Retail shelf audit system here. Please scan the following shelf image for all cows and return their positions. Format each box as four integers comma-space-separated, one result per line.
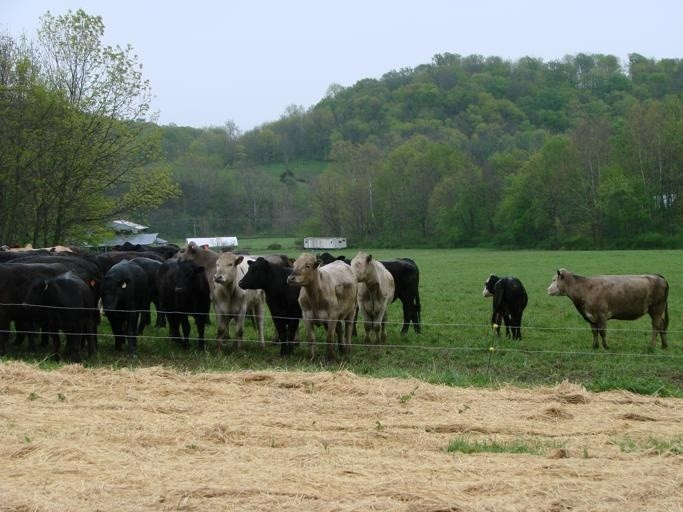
482, 273, 528, 341
547, 267, 669, 353
0, 236, 422, 364
